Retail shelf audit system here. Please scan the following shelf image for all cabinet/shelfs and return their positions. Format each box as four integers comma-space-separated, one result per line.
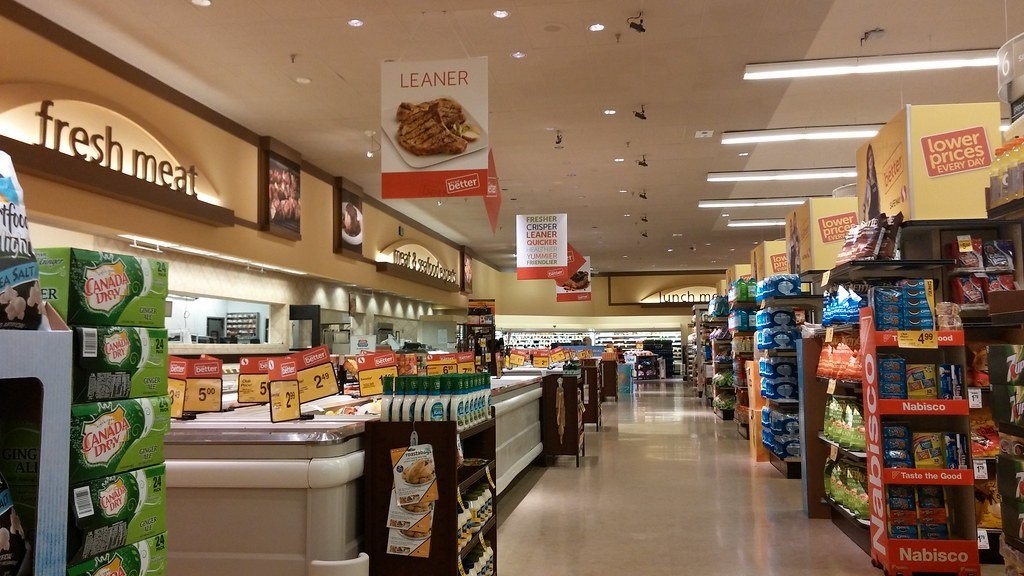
595, 334, 682, 359
685, 197, 1024, 576
225, 312, 259, 339
540, 372, 585, 468
358, 406, 497, 576
563, 364, 602, 432
635, 354, 660, 380
599, 359, 619, 402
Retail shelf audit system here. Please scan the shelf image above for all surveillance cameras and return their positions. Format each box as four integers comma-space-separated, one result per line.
642, 234, 648, 237
642, 218, 648, 223
638, 162, 648, 166
634, 112, 647, 121
631, 22, 646, 33
640, 194, 647, 199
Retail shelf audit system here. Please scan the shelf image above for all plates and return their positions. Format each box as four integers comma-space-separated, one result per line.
398, 529, 431, 540
381, 96, 488, 169
342, 202, 363, 245
399, 505, 431, 514
401, 462, 436, 487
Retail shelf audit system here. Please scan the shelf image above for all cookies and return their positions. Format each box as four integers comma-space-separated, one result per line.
906, 280, 921, 324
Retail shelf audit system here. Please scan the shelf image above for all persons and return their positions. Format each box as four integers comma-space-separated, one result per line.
788, 212, 801, 274
862, 144, 881, 223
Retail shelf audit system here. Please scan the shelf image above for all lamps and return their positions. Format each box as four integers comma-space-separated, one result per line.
634, 154, 648, 167
707, 166, 858, 182
633, 104, 647, 120
697, 194, 832, 210
641, 216, 649, 222
720, 122, 886, 145
632, 104, 646, 120
638, 192, 648, 199
642, 232, 648, 237
742, 46, 999, 82
556, 130, 563, 144
726, 217, 786, 228
627, 10, 647, 35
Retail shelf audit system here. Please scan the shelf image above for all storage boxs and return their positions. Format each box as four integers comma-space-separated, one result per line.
66, 531, 168, 576
998, 535, 1024, 569
991, 384, 1024, 427
67, 462, 167, 567
1004, 558, 1024, 576
70, 395, 171, 484
725, 263, 751, 287
995, 453, 1024, 503
750, 240, 789, 281
855, 102, 1001, 221
784, 197, 859, 275
72, 327, 169, 403
32, 247, 169, 328
716, 280, 726, 297
1000, 495, 1024, 542
986, 343, 1024, 384
331, 331, 349, 354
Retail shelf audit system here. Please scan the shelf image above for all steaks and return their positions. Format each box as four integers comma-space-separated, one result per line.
396, 100, 468, 156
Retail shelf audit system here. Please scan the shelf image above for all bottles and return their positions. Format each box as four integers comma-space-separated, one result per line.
458, 482, 494, 576
380, 371, 492, 432
